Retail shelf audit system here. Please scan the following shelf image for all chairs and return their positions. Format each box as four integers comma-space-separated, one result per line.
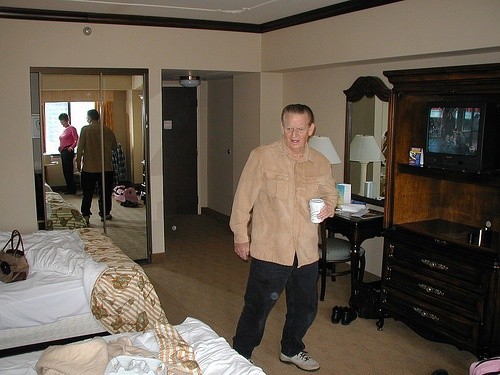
318, 223, 367, 301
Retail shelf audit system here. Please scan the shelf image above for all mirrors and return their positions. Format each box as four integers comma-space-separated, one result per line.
29, 66, 153, 266
337, 75, 392, 206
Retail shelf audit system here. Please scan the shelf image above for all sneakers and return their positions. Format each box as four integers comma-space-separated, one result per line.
279, 351, 321, 371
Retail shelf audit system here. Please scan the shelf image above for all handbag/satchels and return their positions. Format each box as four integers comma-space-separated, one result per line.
0, 230, 30, 284
468, 356, 500, 375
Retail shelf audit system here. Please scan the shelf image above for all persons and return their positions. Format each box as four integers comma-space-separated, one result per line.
76, 109, 117, 225
58, 113, 79, 194
230, 102, 338, 372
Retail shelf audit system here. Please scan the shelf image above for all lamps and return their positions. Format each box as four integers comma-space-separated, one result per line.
350, 134, 385, 196
309, 134, 343, 165
179, 76, 201, 88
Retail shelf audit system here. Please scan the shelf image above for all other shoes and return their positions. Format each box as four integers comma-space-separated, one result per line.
331, 306, 357, 325
62, 188, 74, 194
101, 213, 112, 222
120, 200, 138, 208
83, 215, 90, 224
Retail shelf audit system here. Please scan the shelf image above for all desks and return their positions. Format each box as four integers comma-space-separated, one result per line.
325, 209, 385, 296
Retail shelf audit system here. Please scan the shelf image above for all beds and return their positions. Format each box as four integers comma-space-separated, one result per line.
0, 314, 265, 375
0, 228, 170, 350
44, 191, 89, 230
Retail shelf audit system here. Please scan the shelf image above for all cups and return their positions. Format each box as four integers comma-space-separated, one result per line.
50, 156, 59, 164
309, 198, 325, 224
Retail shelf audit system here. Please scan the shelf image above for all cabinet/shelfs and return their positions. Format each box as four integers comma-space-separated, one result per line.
374, 62, 500, 362
